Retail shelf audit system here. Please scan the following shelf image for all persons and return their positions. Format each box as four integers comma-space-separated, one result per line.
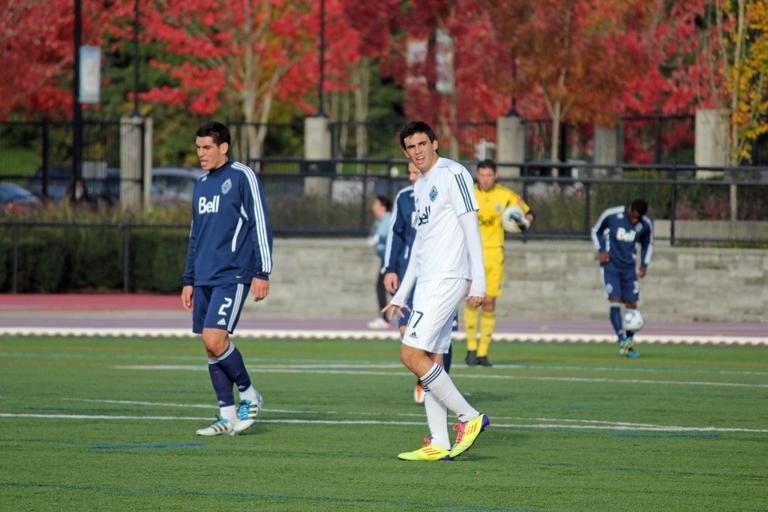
590, 197, 654, 358
383, 153, 453, 405
463, 156, 537, 368
179, 121, 273, 437
380, 120, 492, 462
368, 195, 400, 330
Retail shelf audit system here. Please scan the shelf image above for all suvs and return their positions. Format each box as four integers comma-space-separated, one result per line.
524, 159, 594, 196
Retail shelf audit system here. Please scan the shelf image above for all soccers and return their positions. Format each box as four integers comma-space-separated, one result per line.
622, 308, 646, 334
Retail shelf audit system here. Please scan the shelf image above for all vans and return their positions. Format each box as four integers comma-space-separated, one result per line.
151, 166, 205, 202
31, 167, 121, 200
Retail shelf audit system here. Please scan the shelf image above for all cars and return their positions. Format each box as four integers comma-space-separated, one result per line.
0, 182, 41, 211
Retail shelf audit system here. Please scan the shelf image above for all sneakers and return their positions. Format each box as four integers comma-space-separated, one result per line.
619, 336, 634, 355
413, 381, 425, 404
195, 415, 239, 436
476, 356, 492, 367
625, 349, 641, 359
449, 412, 489, 460
367, 318, 391, 329
465, 351, 480, 365
234, 389, 263, 433
397, 438, 455, 460
